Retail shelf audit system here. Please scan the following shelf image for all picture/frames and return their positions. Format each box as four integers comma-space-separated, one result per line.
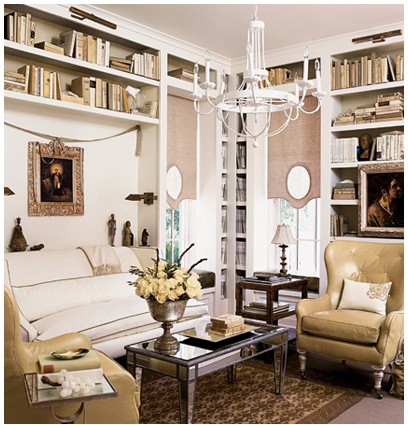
357, 163, 404, 238
27, 142, 84, 216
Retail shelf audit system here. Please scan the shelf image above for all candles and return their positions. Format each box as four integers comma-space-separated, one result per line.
314, 59, 321, 93
205, 48, 211, 82
220, 70, 226, 94
294, 72, 299, 101
303, 45, 311, 81
193, 61, 199, 93
245, 41, 253, 74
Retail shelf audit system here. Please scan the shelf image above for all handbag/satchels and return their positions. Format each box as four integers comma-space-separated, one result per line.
383, 336, 404, 400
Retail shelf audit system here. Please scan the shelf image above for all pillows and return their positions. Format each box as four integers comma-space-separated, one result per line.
336, 278, 392, 316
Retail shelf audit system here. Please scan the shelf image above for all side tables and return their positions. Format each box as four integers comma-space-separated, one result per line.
23, 367, 118, 424
236, 278, 309, 345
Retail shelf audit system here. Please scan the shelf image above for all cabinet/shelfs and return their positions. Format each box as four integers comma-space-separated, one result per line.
221, 63, 254, 314
254, 44, 326, 95
4, 4, 160, 125
161, 40, 222, 101
326, 21, 404, 237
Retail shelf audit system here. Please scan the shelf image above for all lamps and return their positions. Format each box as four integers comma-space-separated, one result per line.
191, 5, 325, 147
272, 223, 296, 280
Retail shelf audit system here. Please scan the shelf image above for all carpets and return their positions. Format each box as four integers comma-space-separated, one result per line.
115, 346, 374, 424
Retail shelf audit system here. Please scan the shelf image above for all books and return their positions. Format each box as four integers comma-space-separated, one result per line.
208, 313, 246, 336
220, 176, 246, 202
243, 299, 289, 314
168, 67, 201, 83
221, 239, 246, 266
223, 143, 246, 167
222, 208, 246, 233
331, 138, 358, 162
266, 69, 292, 86
332, 52, 404, 90
332, 187, 356, 199
330, 214, 348, 235
377, 130, 404, 160
4, 65, 159, 116
222, 275, 242, 295
4, 9, 159, 79
38, 350, 100, 372
333, 91, 404, 125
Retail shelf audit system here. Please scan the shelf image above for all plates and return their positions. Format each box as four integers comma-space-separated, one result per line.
178, 326, 261, 343
50, 348, 89, 360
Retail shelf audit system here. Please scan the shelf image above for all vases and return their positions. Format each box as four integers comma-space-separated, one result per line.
142, 295, 189, 348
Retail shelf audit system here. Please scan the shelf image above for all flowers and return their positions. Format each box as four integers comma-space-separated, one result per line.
126, 242, 207, 303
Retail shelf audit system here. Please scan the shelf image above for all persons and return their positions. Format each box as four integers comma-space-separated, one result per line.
51, 168, 64, 199
124, 221, 132, 247
368, 177, 400, 227
10, 217, 28, 251
108, 214, 117, 247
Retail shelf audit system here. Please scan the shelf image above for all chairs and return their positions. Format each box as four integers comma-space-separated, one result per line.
4, 287, 141, 424
294, 241, 404, 399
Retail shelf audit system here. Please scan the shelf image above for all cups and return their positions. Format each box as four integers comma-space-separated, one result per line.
195, 318, 207, 337
